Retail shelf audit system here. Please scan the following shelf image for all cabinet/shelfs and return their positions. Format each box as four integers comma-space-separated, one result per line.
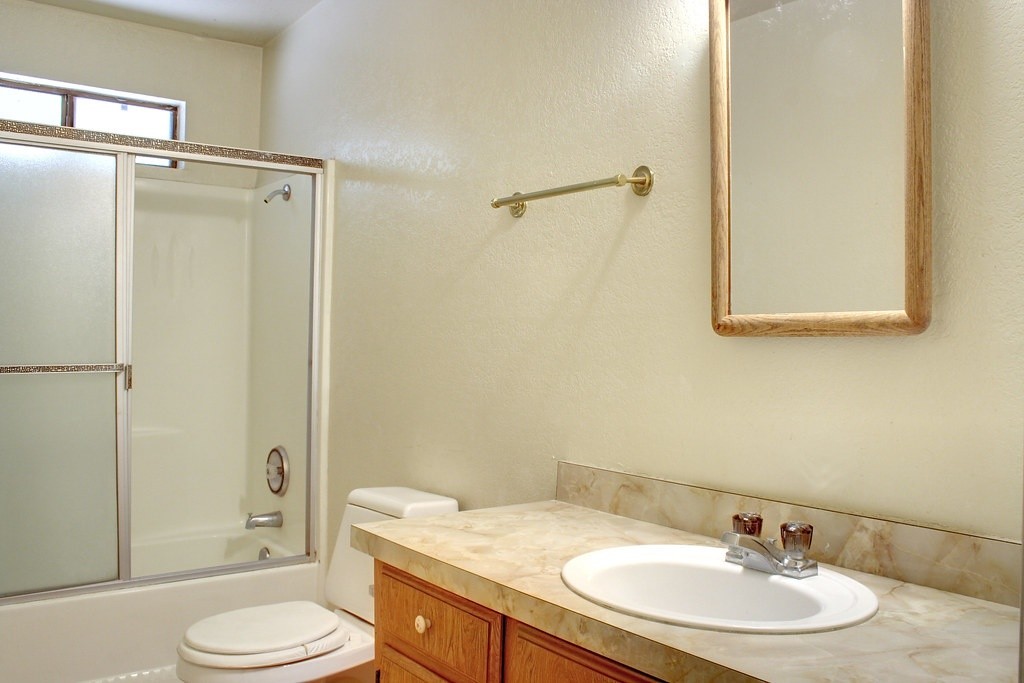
374, 559, 667, 683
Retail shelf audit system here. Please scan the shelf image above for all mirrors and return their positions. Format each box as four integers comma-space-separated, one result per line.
709, 0, 931, 338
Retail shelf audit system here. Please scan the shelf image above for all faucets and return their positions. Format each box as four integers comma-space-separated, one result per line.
245, 510, 283, 529
720, 511, 818, 580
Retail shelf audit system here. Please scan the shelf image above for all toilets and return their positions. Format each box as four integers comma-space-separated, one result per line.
175, 486, 460, 683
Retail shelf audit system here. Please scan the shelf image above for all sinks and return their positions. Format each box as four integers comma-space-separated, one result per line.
560, 545, 880, 634
